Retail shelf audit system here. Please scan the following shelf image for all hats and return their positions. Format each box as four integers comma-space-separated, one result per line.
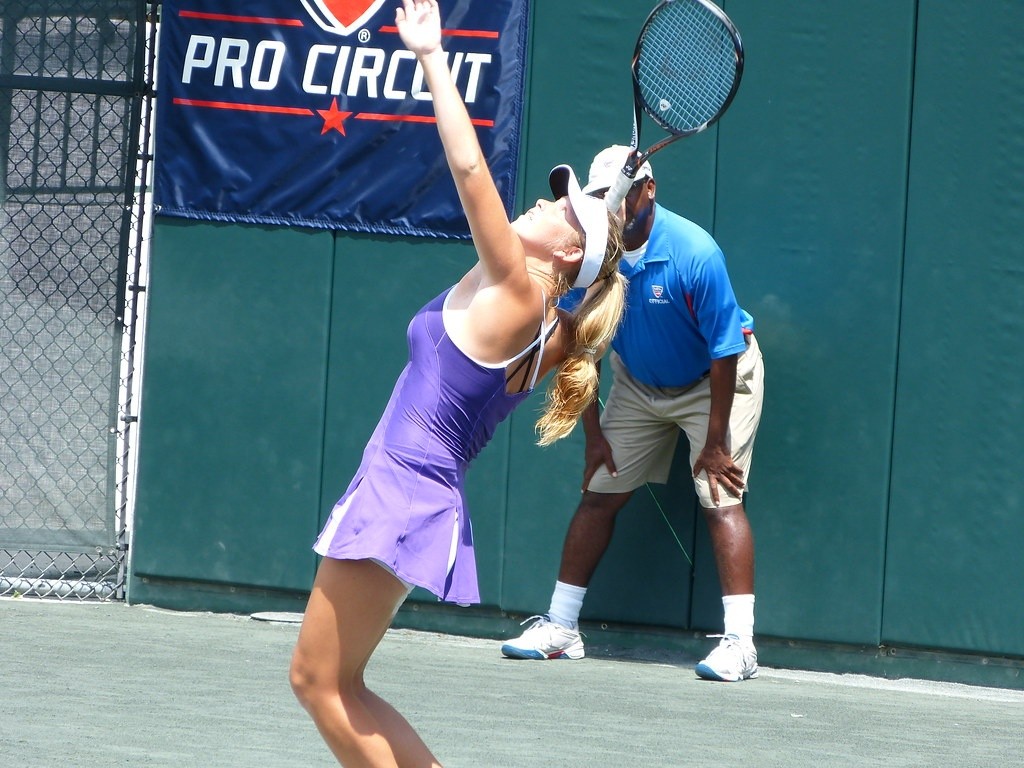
548, 164, 609, 288
582, 144, 653, 194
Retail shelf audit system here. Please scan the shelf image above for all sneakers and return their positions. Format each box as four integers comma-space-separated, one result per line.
501, 616, 586, 660
695, 634, 759, 682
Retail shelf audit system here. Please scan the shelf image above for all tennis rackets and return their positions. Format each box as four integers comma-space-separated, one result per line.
603, 0, 746, 214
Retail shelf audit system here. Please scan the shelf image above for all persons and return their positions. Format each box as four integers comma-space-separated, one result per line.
284, 0, 626, 768
497, 144, 766, 683
